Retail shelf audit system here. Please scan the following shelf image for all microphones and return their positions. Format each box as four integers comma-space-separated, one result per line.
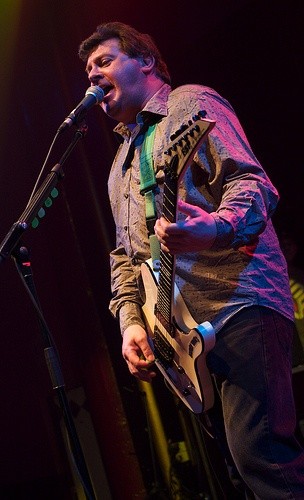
58, 85, 104, 134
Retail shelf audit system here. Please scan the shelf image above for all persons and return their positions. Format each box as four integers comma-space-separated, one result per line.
78, 21, 303, 499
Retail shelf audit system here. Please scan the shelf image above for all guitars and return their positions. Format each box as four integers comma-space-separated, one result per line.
139, 109, 215, 414
